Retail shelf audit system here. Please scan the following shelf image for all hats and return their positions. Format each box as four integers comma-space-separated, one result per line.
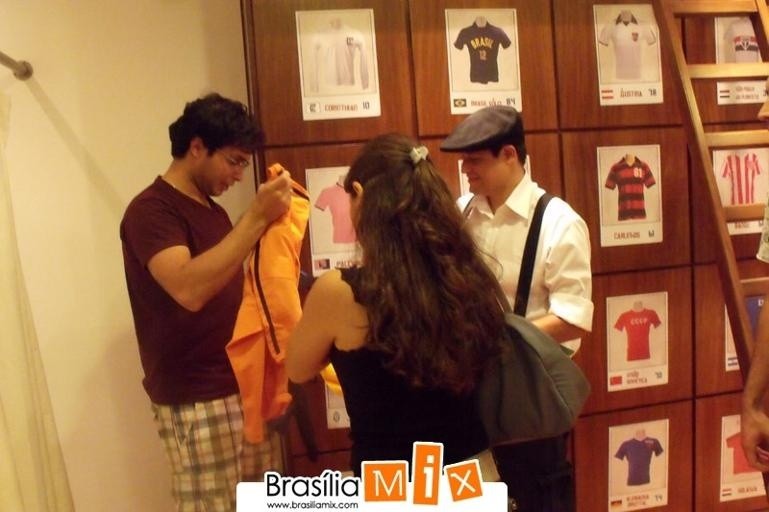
440, 105, 523, 153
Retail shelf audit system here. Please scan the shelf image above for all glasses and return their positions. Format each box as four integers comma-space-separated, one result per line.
217, 148, 250, 167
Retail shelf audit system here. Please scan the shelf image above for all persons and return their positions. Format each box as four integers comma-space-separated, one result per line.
120, 91, 293, 512
740, 76, 769, 472
284, 130, 518, 512
440, 106, 594, 512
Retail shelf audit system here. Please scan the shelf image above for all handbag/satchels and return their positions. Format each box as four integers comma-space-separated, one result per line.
476, 313, 591, 442
